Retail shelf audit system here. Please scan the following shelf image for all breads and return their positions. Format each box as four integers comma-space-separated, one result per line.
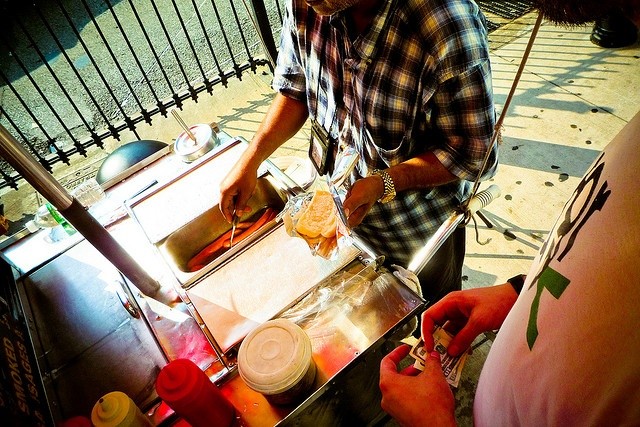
296, 187, 337, 238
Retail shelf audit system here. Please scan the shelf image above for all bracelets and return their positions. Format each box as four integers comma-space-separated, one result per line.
507, 273, 526, 297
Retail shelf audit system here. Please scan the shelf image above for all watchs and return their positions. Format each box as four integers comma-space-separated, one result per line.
366, 168, 398, 206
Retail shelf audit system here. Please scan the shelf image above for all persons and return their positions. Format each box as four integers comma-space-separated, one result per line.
217, 1, 501, 341
378, 0, 639, 426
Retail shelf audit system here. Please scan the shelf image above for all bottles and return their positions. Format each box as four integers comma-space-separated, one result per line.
42, 204, 105, 245
155, 358, 236, 425
91, 389, 151, 426
24, 177, 107, 233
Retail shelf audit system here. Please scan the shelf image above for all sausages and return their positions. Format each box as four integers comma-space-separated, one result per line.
185, 228, 242, 272
223, 204, 280, 249
